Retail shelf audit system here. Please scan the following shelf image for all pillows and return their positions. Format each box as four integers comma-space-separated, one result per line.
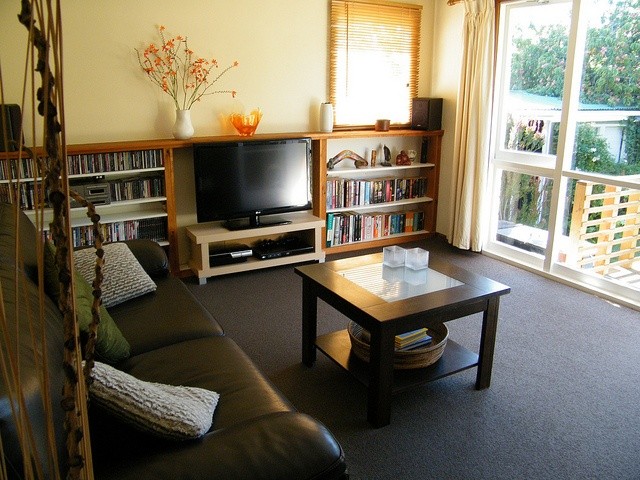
73, 242, 158, 309
44, 237, 133, 364
75, 360, 221, 442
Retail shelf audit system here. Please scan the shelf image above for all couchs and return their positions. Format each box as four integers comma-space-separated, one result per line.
1, 192, 349, 479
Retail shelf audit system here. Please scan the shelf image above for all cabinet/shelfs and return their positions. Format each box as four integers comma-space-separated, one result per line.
313, 129, 444, 255
1, 139, 181, 281
184, 213, 326, 285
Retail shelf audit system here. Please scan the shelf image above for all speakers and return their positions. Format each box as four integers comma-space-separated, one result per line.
411, 97, 443, 129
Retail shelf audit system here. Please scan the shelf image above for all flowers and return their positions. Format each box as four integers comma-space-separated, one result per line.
134, 24, 239, 110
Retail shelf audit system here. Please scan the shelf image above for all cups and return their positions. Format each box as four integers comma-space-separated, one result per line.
406, 247, 430, 271
375, 120, 391, 131
382, 265, 405, 284
405, 266, 428, 286
383, 245, 406, 268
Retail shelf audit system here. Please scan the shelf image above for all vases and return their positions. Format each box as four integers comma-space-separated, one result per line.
320, 103, 334, 132
171, 110, 194, 139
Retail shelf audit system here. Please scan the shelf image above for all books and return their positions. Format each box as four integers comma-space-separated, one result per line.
326, 211, 425, 247
326, 176, 428, 209
0, 149, 163, 180
39, 217, 168, 248
395, 327, 433, 352
0, 175, 165, 210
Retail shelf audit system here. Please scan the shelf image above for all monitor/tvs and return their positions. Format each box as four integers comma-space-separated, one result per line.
191, 137, 313, 232
